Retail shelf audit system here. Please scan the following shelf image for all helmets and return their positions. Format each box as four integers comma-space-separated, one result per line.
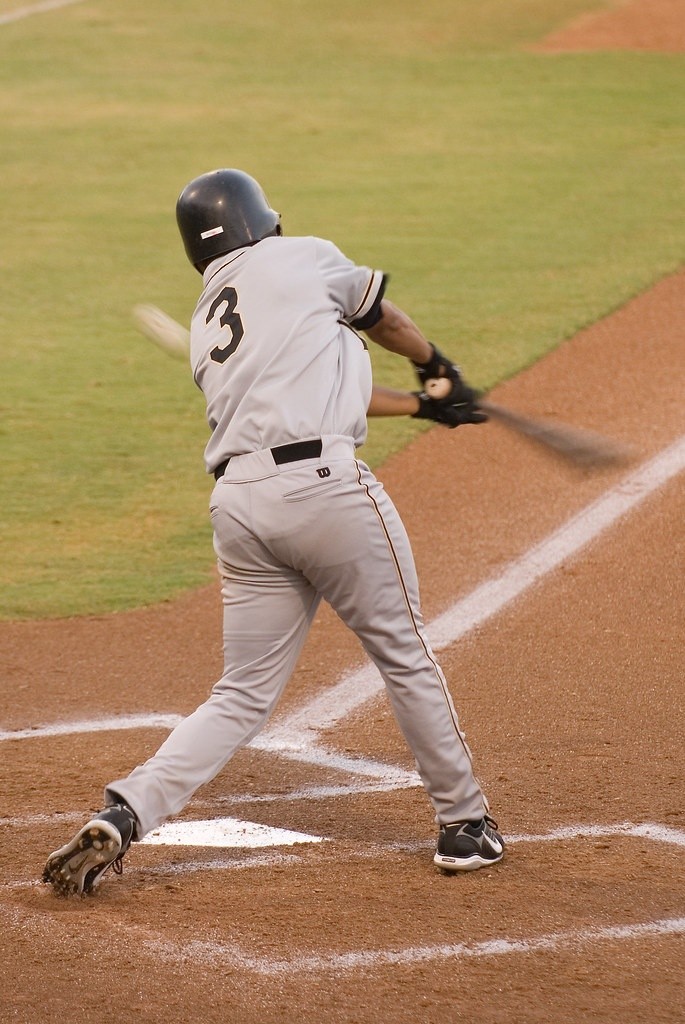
177, 168, 282, 275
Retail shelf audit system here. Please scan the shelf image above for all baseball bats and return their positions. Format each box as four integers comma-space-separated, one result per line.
425, 373, 620, 475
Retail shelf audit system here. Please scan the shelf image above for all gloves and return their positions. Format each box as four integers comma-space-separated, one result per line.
411, 343, 488, 427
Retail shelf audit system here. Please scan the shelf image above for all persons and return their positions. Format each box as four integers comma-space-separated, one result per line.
42, 167, 507, 898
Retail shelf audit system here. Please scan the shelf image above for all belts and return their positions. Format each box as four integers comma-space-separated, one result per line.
214, 440, 322, 481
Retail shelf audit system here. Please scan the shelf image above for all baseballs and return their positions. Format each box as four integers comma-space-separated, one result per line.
134, 303, 189, 363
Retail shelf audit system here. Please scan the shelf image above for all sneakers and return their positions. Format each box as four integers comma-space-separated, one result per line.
434, 814, 505, 870
41, 803, 136, 897
